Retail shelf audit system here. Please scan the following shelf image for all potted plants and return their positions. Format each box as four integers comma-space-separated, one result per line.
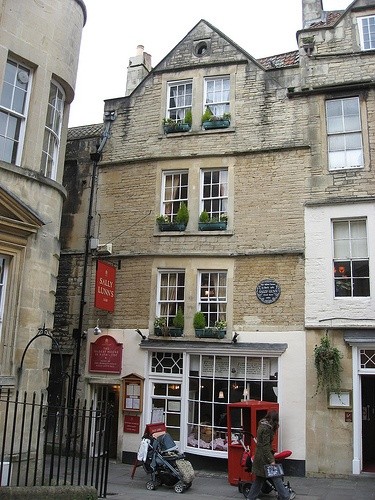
312, 336, 346, 405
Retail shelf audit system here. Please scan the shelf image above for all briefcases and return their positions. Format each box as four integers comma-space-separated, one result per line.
264, 463, 285, 478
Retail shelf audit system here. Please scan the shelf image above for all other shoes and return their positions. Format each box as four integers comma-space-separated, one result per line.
289, 492, 296, 500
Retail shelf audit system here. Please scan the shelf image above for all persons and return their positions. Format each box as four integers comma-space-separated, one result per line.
249, 412, 290, 500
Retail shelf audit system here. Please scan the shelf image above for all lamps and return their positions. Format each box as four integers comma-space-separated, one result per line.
94, 319, 101, 335
232, 332, 239, 343
135, 328, 146, 338
81, 330, 87, 338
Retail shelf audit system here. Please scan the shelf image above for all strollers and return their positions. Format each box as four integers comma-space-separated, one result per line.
238, 431, 296, 500
140, 434, 195, 493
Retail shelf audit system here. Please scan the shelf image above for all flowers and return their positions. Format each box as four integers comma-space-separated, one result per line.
201, 105, 231, 122
156, 201, 189, 225
155, 305, 184, 339
199, 209, 227, 224
162, 109, 194, 129
336, 275, 354, 293
194, 311, 226, 340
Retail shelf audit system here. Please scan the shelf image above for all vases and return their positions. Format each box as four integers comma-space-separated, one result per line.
154, 327, 183, 336
204, 121, 230, 129
160, 222, 186, 231
164, 123, 190, 133
196, 329, 225, 338
199, 222, 227, 230
